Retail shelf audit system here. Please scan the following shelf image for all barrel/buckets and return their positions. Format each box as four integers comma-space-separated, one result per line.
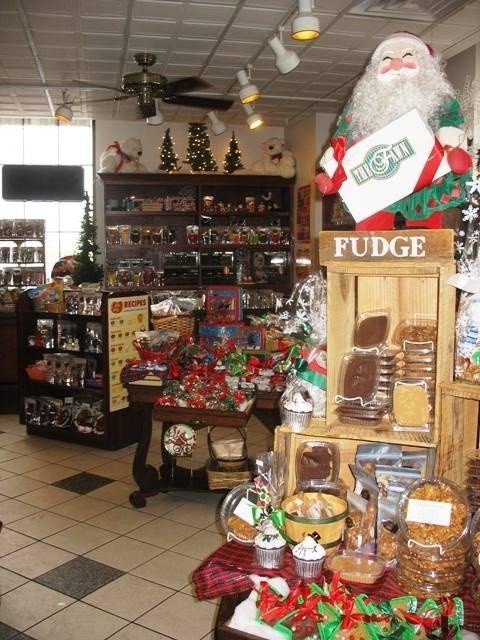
280, 492, 347, 566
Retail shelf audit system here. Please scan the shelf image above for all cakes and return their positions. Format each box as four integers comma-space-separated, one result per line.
283, 391, 313, 413
254, 519, 286, 550
292, 531, 326, 562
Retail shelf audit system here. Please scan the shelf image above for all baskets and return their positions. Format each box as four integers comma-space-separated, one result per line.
206, 456, 255, 490
150, 315, 195, 335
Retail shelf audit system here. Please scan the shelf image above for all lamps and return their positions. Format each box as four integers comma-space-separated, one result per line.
53, 87, 265, 135
240, 0, 328, 98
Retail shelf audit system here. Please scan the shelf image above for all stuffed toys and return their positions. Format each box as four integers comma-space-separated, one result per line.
97, 136, 148, 173
250, 136, 296, 180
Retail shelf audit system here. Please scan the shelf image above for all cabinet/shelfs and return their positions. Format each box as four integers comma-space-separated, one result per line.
268, 229, 479, 566
1, 218, 47, 415
98, 169, 296, 326
17, 287, 154, 451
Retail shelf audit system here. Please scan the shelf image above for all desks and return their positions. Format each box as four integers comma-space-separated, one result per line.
202, 518, 480, 640
124, 373, 285, 510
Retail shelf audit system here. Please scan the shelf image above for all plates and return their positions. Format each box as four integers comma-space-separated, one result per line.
162, 424, 197, 457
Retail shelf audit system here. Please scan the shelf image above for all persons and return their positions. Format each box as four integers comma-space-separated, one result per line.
314, 29, 474, 233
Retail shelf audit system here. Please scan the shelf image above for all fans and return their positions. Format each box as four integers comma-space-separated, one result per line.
57, 51, 238, 120
